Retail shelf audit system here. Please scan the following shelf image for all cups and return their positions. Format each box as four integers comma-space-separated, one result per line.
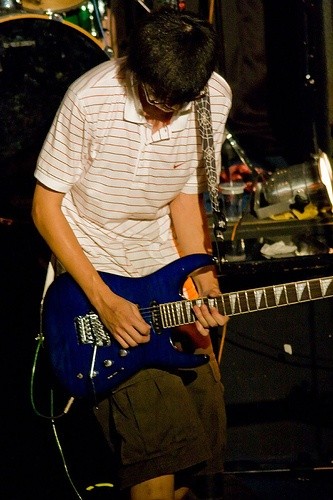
219, 183, 246, 223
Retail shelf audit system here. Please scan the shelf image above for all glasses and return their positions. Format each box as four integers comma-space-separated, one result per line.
139, 78, 208, 106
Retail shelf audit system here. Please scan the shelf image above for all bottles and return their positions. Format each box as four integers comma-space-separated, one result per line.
253, 160, 323, 221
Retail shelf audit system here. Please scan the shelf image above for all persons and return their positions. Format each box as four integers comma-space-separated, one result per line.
32, 5, 232, 500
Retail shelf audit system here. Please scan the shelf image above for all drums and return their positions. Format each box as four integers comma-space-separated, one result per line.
1, 15, 115, 223
20, 0, 93, 35
21, 0, 87, 13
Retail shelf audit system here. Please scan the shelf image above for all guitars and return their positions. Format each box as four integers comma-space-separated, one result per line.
41, 252, 333, 402
